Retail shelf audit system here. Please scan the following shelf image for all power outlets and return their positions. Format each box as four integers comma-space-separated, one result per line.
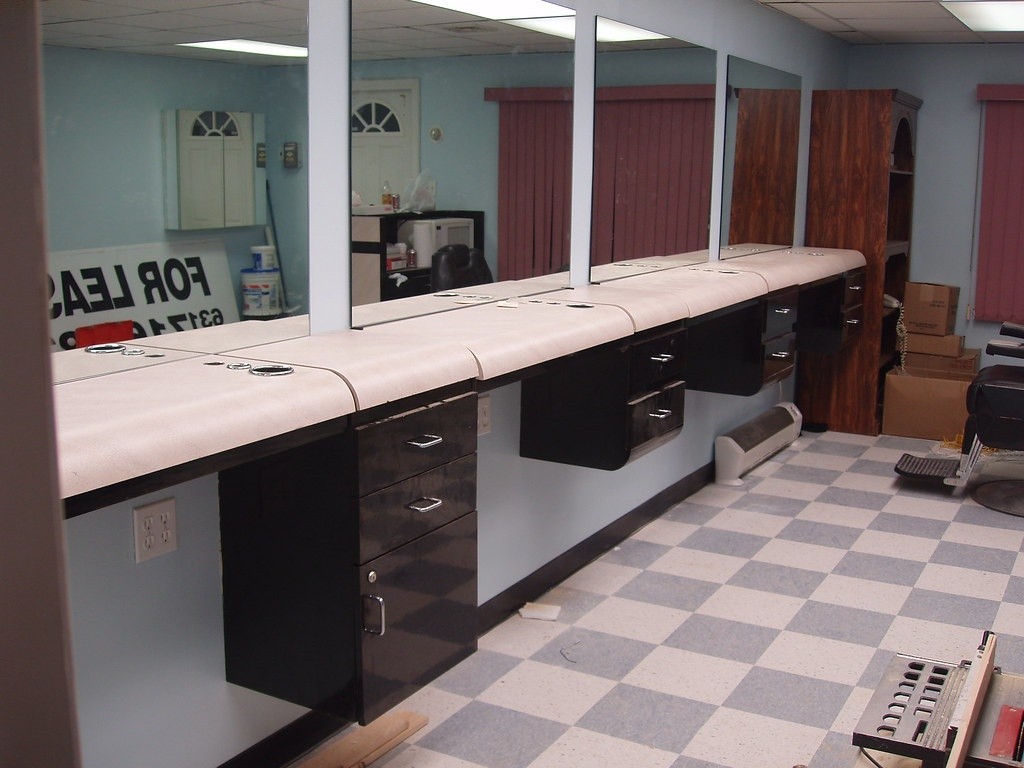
478, 394, 492, 437
132, 496, 177, 564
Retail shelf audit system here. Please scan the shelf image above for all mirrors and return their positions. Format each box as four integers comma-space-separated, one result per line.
345, 0, 577, 329
35, 0, 311, 386
589, 15, 718, 283
719, 54, 801, 260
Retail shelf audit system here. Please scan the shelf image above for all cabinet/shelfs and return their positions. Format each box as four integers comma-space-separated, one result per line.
352, 209, 486, 307
218, 391, 478, 725
684, 288, 797, 397
161, 109, 256, 231
792, 89, 922, 436
520, 321, 685, 471
796, 268, 865, 350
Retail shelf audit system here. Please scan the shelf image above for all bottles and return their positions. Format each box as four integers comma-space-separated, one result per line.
381, 181, 391, 205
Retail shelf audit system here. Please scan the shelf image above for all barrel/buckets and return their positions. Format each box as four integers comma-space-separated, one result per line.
240, 267, 282, 320
250, 245, 275, 270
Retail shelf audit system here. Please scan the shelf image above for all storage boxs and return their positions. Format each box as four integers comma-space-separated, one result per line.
881, 366, 970, 441
902, 348, 981, 381
902, 281, 960, 335
894, 333, 966, 357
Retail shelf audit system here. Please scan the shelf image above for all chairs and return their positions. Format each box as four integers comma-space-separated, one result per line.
894, 321, 1024, 517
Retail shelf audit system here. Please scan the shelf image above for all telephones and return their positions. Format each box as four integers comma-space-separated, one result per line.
882, 293, 900, 308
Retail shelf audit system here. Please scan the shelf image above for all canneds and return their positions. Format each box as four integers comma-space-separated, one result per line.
391, 193, 400, 210
407, 248, 417, 268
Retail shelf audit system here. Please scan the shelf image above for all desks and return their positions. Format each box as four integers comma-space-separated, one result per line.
52, 241, 867, 519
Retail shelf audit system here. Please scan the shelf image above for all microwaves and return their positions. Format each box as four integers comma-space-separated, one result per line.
397, 218, 475, 268
